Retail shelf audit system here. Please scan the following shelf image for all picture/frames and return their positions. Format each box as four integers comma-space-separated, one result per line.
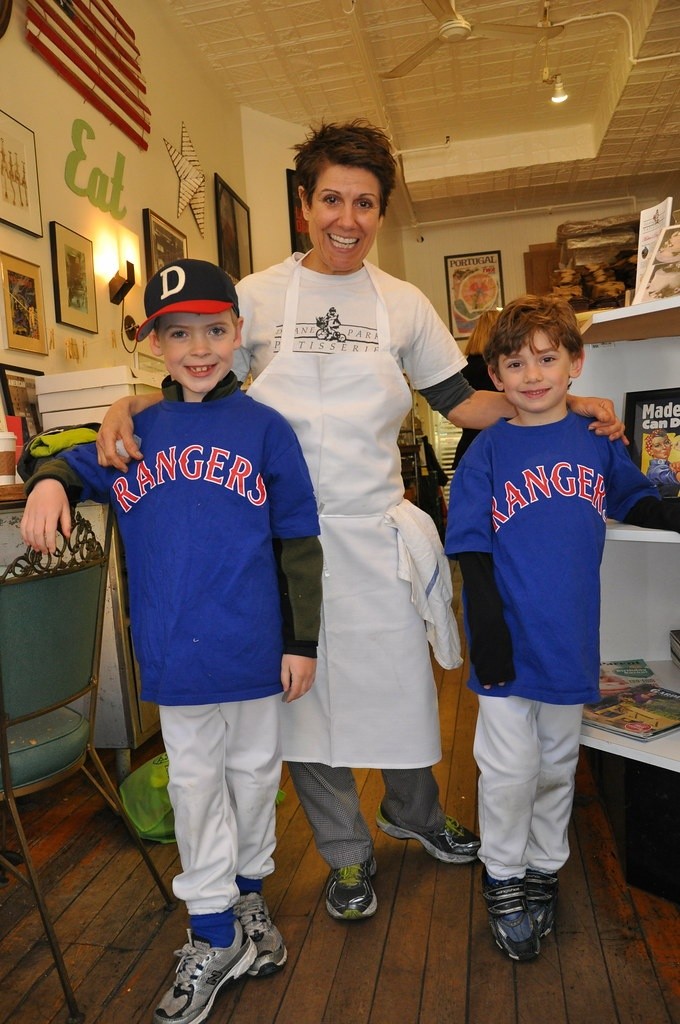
0, 362, 45, 444
0, 109, 43, 239
143, 208, 189, 283
286, 168, 314, 254
214, 172, 253, 286
0, 249, 49, 356
49, 220, 99, 335
444, 250, 505, 341
631, 224, 680, 305
623, 387, 680, 499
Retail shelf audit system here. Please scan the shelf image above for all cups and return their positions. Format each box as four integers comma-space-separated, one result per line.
0, 432, 17, 485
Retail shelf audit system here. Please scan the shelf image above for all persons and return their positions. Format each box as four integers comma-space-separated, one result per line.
19, 259, 326, 1024
446, 293, 680, 964
616, 688, 659, 705
97, 114, 630, 921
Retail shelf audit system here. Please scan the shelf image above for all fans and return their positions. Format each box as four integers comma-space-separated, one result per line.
379, 0, 565, 81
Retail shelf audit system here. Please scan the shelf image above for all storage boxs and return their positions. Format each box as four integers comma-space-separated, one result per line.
37, 384, 162, 415
33, 364, 163, 396
42, 406, 110, 432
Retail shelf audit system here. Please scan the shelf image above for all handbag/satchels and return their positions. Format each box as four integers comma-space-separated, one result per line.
120, 752, 287, 843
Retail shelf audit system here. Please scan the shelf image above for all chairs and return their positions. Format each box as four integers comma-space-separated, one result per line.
0, 503, 179, 1024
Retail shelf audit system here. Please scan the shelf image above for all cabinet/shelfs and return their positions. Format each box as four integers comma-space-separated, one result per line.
397, 371, 419, 513
573, 295, 680, 911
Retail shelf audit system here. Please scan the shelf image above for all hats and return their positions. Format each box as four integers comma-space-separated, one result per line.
135, 257, 240, 343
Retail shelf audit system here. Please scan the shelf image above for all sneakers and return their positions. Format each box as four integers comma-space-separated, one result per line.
376, 802, 480, 862
233, 891, 287, 976
325, 855, 377, 920
481, 869, 558, 963
152, 919, 257, 1024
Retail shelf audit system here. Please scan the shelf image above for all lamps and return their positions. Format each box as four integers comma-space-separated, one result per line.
542, 66, 570, 104
108, 261, 135, 306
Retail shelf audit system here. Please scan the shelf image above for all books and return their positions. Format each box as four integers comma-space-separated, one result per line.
582, 195, 680, 744
